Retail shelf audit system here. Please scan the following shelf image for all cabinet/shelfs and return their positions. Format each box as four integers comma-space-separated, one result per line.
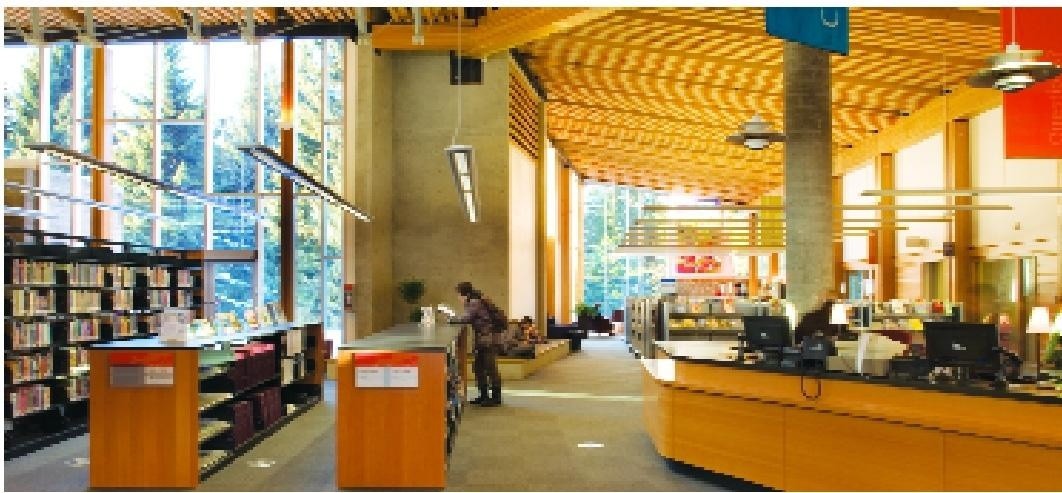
80, 322, 325, 491
3, 242, 204, 459
339, 321, 475, 491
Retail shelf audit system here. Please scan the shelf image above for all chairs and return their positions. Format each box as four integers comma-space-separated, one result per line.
548, 314, 586, 351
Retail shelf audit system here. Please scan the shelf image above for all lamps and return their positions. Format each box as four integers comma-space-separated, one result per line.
960, 7, 1060, 96
233, 137, 379, 227
725, 113, 791, 151
22, 138, 226, 210
440, 6, 484, 228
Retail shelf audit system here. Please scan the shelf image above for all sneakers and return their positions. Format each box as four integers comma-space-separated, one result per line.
471, 397, 500, 406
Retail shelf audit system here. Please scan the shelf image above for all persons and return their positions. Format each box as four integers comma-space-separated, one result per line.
444, 283, 509, 407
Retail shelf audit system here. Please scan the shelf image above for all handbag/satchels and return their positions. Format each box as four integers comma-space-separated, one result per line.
481, 299, 508, 332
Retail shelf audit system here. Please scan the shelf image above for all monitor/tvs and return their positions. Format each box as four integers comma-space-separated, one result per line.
924, 322, 998, 386
743, 316, 791, 365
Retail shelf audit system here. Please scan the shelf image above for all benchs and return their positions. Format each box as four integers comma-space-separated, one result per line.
463, 337, 570, 381
